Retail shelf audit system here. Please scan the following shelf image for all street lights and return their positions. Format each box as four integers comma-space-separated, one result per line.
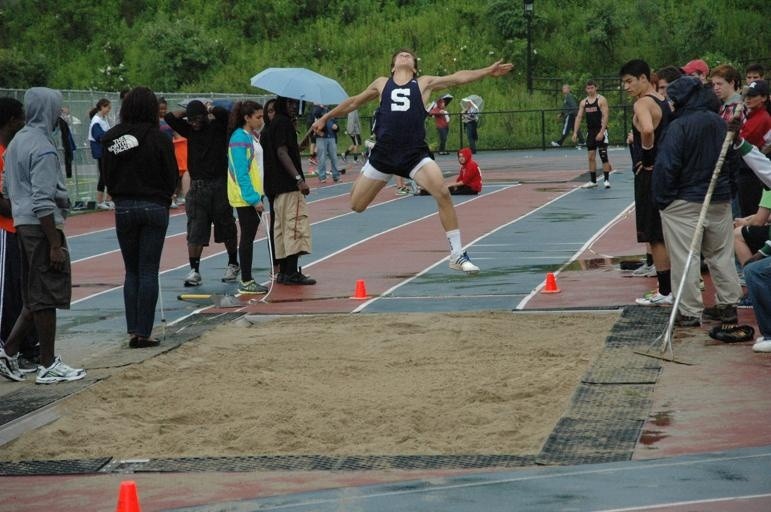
523, 0, 537, 94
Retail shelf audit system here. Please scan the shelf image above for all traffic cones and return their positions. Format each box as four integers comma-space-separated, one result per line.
117, 479, 141, 510
352, 279, 372, 302
541, 270, 562, 296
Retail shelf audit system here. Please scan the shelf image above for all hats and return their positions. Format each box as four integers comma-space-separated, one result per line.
187, 100, 207, 117
353, 159, 363, 165
680, 59, 710, 77
745, 79, 771, 97
320, 178, 326, 183
333, 178, 344, 183
341, 152, 346, 162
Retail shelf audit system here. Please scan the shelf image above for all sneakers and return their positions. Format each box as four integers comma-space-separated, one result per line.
635, 291, 674, 306
632, 263, 657, 277
394, 183, 423, 196
752, 335, 771, 353
736, 295, 754, 309
448, 254, 480, 273
271, 270, 316, 285
576, 141, 586, 149
184, 271, 202, 286
604, 180, 611, 189
698, 274, 704, 290
34, 360, 87, 384
238, 280, 269, 295
703, 305, 738, 322
551, 141, 561, 147
97, 200, 114, 210
221, 264, 240, 283
581, 181, 598, 189
709, 324, 755, 342
0, 343, 38, 382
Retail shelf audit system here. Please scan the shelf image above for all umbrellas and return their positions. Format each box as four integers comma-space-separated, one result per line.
426, 93, 454, 114
252, 66, 354, 105
460, 94, 486, 118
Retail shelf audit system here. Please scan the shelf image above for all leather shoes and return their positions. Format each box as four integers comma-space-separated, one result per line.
127, 335, 160, 348
679, 311, 701, 327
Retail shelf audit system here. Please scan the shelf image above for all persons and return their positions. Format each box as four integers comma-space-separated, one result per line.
89, 86, 181, 346
158, 95, 341, 294
551, 83, 587, 146
343, 106, 362, 166
458, 102, 479, 153
619, 54, 771, 353
414, 147, 482, 196
51, 107, 81, 182
1, 97, 42, 371
313, 49, 514, 270
0, 86, 86, 384
572, 81, 614, 189
396, 173, 412, 195
430, 101, 451, 154
